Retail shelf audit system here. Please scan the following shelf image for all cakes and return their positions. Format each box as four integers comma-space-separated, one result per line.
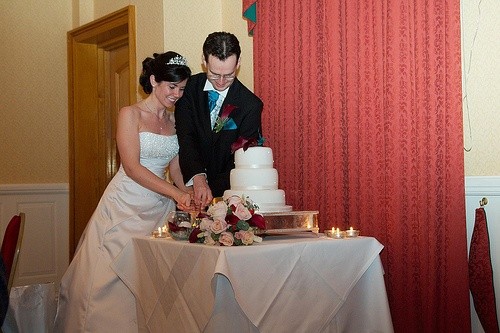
222, 146, 292, 212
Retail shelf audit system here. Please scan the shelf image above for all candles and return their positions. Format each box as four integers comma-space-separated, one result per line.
158, 225, 168, 237
332, 227, 353, 237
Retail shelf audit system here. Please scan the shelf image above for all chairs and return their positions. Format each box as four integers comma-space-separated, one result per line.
0, 213, 26, 332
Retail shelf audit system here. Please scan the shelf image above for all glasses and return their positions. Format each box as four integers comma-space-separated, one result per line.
206, 63, 236, 82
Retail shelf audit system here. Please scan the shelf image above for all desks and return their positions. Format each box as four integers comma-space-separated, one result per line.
133, 232, 394, 333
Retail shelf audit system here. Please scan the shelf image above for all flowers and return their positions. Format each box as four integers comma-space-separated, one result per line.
214, 104, 239, 133
168, 194, 266, 246
229, 128, 265, 155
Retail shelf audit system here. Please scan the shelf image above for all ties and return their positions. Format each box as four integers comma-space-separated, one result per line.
207, 90, 220, 113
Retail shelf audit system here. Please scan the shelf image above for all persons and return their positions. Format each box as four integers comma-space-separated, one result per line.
172, 31, 264, 211
53, 51, 203, 333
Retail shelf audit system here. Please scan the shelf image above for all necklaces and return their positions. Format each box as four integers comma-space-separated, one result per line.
143, 100, 166, 130
